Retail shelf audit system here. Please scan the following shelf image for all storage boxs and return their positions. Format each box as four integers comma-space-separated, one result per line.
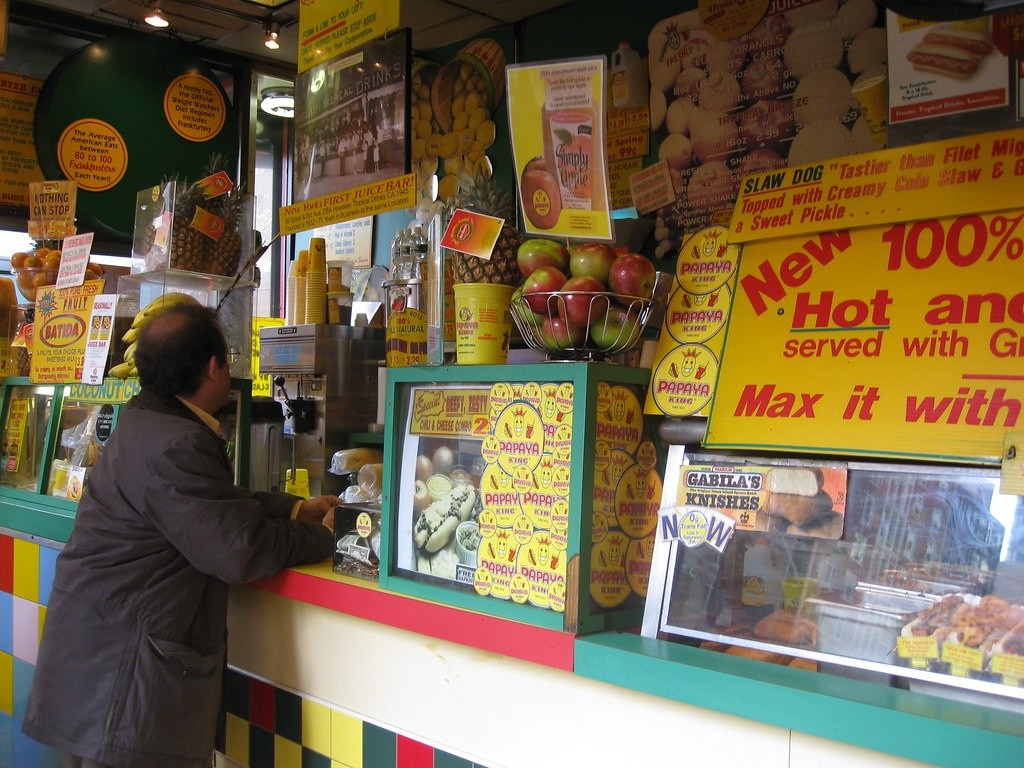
130, 183, 254, 292
108, 272, 254, 379
333, 502, 382, 582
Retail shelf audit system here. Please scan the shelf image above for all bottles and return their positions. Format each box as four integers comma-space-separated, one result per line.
327, 292, 356, 326
392, 228, 410, 279
328, 260, 354, 291
409, 227, 427, 279
611, 42, 646, 109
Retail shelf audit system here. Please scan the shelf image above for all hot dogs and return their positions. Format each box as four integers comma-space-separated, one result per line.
906, 27, 993, 80
414, 484, 476, 553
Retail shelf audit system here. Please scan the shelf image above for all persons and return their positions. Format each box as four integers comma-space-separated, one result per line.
20, 300, 347, 767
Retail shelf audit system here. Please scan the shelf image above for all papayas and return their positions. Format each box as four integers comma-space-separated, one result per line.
0, 277, 20, 341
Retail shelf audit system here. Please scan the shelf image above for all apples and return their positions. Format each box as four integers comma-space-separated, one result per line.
520, 156, 561, 231
736, 14, 791, 213
510, 238, 655, 352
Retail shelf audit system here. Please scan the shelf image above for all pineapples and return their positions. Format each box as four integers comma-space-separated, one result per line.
143, 152, 250, 276
449, 169, 524, 285
16, 308, 35, 376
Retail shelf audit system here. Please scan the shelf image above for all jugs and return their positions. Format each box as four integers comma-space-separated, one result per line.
250, 395, 286, 493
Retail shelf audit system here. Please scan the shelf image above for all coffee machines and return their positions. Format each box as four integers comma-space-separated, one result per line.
260, 325, 387, 494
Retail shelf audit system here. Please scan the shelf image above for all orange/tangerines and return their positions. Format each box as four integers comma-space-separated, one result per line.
11, 249, 101, 302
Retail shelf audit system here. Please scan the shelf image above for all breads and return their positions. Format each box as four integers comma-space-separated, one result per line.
763, 466, 833, 526
330, 447, 385, 506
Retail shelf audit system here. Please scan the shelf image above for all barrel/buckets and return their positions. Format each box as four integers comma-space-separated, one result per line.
411, 55, 444, 84
431, 37, 507, 132
451, 283, 518, 364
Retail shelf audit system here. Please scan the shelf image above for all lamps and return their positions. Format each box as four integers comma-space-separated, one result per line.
264, 23, 281, 50
144, 0, 169, 28
261, 86, 295, 117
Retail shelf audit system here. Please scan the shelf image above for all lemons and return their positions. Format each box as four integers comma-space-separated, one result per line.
409, 63, 495, 199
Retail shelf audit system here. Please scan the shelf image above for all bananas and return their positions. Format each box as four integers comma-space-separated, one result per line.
107, 292, 201, 376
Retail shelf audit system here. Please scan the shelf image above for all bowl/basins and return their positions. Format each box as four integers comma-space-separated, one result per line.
11, 267, 102, 302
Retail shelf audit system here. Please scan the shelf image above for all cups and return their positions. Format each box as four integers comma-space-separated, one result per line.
328, 447, 382, 503
852, 75, 889, 144
295, 250, 308, 324
286, 260, 295, 326
53, 464, 70, 495
541, 100, 606, 213
285, 468, 311, 499
781, 577, 820, 611
456, 521, 481, 565
89, 316, 102, 340
426, 474, 454, 503
304, 238, 326, 324
448, 465, 473, 487
100, 316, 112, 339
